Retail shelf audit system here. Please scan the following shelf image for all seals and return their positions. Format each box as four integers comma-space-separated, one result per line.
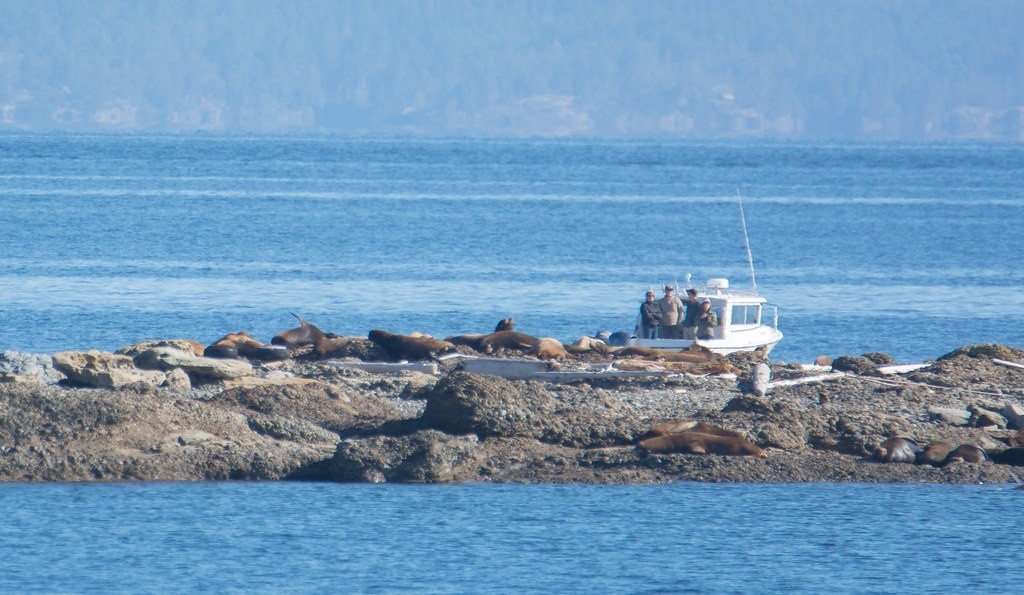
189, 310, 744, 377
636, 420, 1024, 469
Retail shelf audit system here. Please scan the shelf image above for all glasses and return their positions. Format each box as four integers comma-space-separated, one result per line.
688, 293, 691, 295
648, 295, 654, 298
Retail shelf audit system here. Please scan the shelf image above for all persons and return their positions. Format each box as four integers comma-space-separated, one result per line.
640, 286, 718, 340
495, 318, 513, 332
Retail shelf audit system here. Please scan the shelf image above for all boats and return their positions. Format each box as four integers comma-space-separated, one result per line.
627, 186, 784, 354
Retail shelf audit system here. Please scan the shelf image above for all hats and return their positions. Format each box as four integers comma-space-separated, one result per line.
702, 297, 710, 303
686, 289, 698, 297
665, 286, 674, 292
